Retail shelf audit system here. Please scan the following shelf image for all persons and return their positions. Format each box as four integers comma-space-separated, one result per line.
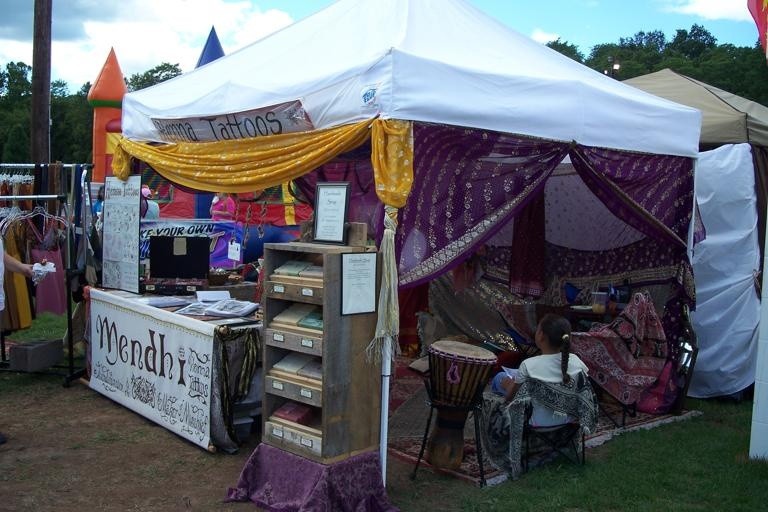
139, 187, 161, 260
92, 185, 106, 222
0, 237, 35, 368
489, 313, 589, 454
209, 191, 236, 220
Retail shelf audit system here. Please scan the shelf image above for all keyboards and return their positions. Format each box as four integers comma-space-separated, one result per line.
146, 284, 198, 295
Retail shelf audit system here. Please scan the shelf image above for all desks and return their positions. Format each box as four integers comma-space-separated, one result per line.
83, 286, 263, 453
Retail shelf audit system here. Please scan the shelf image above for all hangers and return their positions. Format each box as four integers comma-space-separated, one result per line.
0, 164, 35, 186
0, 195, 70, 235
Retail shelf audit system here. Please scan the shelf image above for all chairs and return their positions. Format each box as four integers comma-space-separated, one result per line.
473, 372, 589, 470
569, 290, 666, 423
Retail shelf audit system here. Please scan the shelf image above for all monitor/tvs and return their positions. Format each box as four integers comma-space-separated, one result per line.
150, 236, 210, 280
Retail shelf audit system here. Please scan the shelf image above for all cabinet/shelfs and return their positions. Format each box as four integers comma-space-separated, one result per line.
261, 242, 379, 466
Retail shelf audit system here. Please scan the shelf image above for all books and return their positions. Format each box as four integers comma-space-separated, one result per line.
273, 259, 312, 277
296, 359, 322, 382
145, 290, 260, 317
297, 306, 324, 332
272, 302, 317, 326
272, 352, 319, 375
299, 264, 323, 280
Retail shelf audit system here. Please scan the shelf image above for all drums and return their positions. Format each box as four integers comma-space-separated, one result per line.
423, 338, 499, 470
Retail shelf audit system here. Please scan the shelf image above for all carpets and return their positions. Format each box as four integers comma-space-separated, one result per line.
387, 351, 702, 488
